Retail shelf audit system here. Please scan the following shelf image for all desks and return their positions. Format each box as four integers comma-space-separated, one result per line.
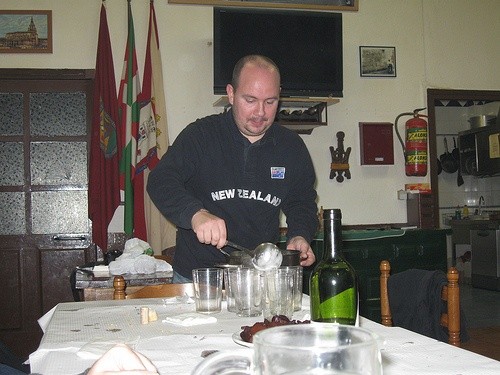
278, 228, 452, 323
22, 297, 500, 375
75, 269, 191, 302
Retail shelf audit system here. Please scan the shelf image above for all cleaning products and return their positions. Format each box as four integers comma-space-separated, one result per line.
463, 205, 469, 218
454, 205, 461, 224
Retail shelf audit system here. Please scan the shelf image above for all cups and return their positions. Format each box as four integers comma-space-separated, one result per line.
252, 322, 384, 375
192, 264, 304, 322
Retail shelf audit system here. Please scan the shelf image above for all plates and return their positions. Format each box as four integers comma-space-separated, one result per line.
231, 330, 254, 348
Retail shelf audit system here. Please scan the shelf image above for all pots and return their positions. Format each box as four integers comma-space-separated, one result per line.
440, 138, 458, 172
475, 115, 495, 127
229, 249, 302, 266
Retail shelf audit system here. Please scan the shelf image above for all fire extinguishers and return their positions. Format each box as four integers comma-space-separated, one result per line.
395, 107, 429, 177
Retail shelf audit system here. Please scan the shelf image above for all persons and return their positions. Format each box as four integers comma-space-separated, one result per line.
146, 56, 318, 284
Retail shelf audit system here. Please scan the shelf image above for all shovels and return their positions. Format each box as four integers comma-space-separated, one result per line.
439, 138, 458, 174
456, 158, 464, 187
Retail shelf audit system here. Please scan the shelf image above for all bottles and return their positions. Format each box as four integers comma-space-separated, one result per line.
310, 208, 360, 328
463, 205, 470, 220
455, 205, 461, 220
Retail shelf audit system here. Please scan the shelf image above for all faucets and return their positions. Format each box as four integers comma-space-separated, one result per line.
477, 196, 485, 217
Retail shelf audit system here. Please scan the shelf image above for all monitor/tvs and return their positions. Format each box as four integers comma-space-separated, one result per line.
211, 6, 345, 97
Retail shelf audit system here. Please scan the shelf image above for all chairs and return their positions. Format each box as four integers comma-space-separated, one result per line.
380, 261, 460, 347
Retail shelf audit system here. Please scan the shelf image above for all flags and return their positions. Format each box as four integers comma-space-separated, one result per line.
138, 0, 176, 260
117, 4, 141, 238
88, 4, 120, 254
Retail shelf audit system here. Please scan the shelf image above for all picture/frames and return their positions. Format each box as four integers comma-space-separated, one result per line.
359, 46, 397, 77
0, 10, 53, 53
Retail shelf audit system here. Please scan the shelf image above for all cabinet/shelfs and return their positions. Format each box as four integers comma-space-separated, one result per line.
457, 124, 500, 178
212, 96, 341, 135
